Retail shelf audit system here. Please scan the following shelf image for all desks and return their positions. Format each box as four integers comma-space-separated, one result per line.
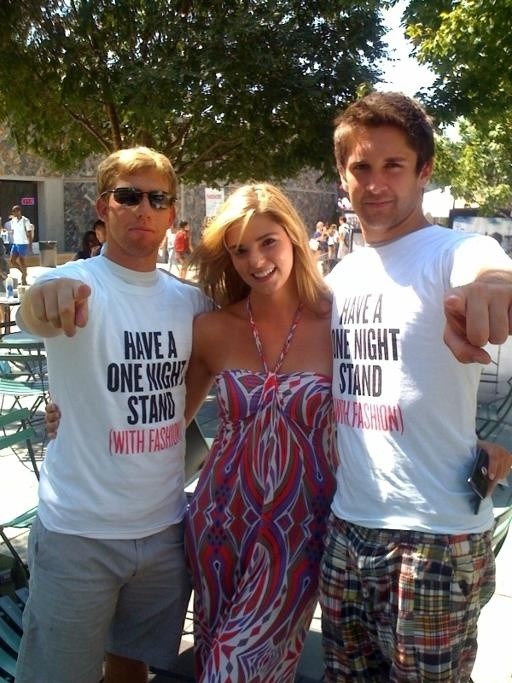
1, 332, 48, 381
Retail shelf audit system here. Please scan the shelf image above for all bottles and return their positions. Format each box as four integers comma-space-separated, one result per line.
6, 274, 13, 300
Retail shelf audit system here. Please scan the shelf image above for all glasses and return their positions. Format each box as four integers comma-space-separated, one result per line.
100, 188, 178, 209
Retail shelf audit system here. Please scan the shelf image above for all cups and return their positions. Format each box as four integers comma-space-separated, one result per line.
17, 288, 25, 302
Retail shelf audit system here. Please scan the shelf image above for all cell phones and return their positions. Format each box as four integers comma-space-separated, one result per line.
468, 448, 491, 514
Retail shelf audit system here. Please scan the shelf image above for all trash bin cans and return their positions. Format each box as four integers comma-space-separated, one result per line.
39, 240, 57, 267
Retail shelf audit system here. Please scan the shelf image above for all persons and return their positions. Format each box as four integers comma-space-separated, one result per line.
11, 144, 222, 682
71, 219, 108, 261
308, 215, 352, 264
0, 205, 35, 342
324, 90, 511, 682
159, 220, 194, 279
41, 181, 512, 683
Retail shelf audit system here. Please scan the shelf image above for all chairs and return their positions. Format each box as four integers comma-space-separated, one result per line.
475, 344, 512, 441
0, 354, 50, 579
